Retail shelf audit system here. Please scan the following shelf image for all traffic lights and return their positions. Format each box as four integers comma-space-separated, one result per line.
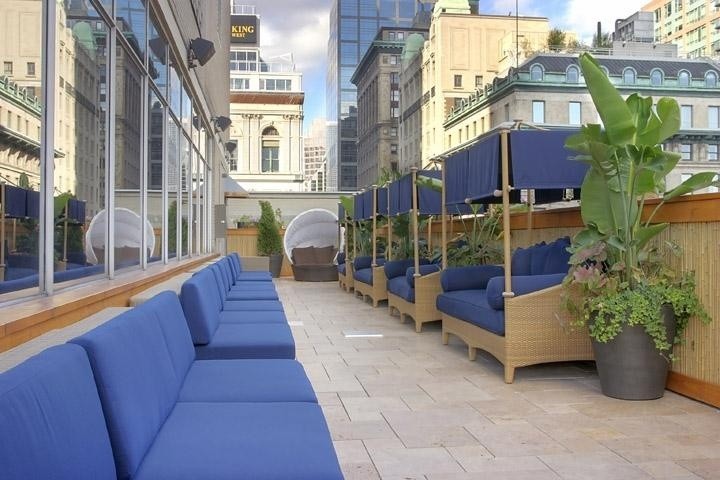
313, 246, 333, 264
293, 246, 316, 265
331, 249, 338, 263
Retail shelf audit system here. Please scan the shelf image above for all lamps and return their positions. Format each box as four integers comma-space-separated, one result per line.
193, 115, 202, 131
0, 182, 184, 295
284, 208, 345, 282
210, 115, 232, 135
149, 38, 166, 64
225, 142, 236, 164
188, 37, 216, 71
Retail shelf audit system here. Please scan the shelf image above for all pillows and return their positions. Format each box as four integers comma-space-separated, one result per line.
331, 249, 338, 263
313, 246, 333, 264
293, 246, 316, 265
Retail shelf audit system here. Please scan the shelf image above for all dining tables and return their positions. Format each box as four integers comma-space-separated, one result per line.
555, 53, 720, 401
258, 200, 284, 278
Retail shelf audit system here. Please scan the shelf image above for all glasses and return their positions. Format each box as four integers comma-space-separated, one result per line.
210, 115, 232, 135
193, 115, 202, 131
149, 38, 166, 64
188, 37, 216, 71
225, 142, 236, 164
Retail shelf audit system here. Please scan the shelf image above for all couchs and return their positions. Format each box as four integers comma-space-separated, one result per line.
0, 252, 345, 480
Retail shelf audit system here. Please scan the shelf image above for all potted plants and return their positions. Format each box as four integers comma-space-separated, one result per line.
555, 53, 720, 401
258, 200, 284, 278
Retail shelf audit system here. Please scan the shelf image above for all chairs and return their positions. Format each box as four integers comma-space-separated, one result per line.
0, 182, 184, 295
338, 128, 596, 384
284, 208, 345, 282
